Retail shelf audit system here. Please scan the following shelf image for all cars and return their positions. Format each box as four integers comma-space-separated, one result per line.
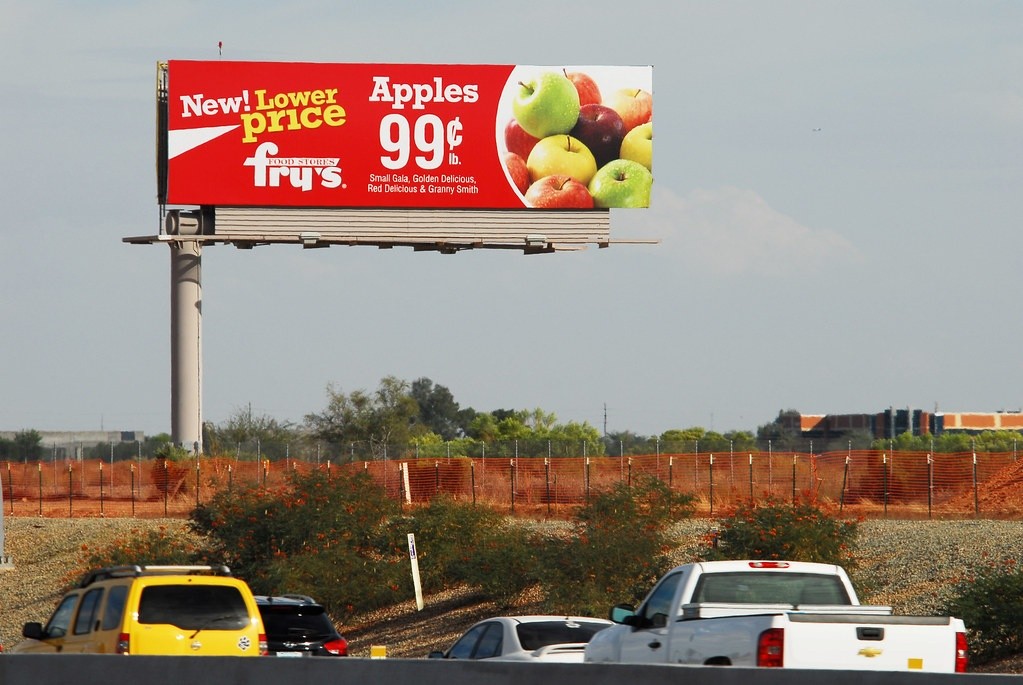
427, 615, 620, 662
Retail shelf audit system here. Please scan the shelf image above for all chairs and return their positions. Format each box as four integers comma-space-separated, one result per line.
706, 578, 735, 603
800, 580, 840, 604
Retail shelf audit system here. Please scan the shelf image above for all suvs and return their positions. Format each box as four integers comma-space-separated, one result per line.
246, 595, 349, 656
12, 568, 268, 656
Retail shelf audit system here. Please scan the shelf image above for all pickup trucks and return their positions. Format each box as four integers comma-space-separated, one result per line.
586, 560, 967, 672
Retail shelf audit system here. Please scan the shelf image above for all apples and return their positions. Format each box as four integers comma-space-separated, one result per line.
502, 67, 652, 209
